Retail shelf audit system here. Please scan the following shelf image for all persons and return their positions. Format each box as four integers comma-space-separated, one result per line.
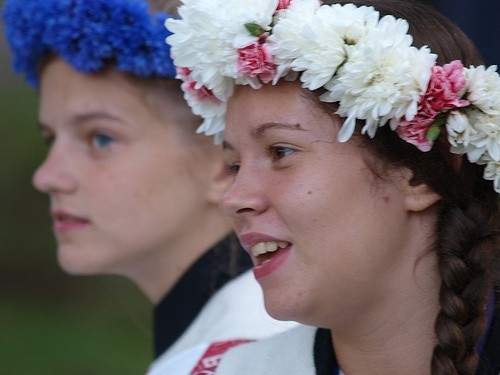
162, 0, 500, 375
0, 0, 303, 375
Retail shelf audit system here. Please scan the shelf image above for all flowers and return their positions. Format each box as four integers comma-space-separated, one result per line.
0, 1, 179, 84
162, 0, 499, 193
